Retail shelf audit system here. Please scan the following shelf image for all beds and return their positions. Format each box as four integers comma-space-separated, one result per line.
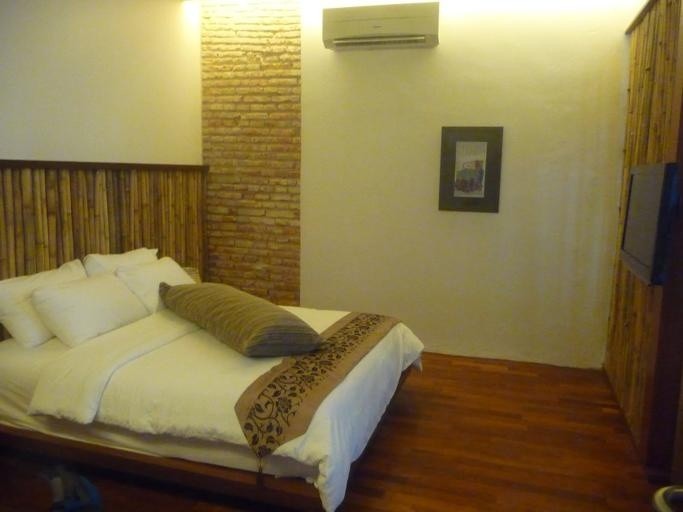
0, 160, 425, 512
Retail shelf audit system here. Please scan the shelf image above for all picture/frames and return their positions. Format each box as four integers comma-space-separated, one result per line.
438, 127, 503, 212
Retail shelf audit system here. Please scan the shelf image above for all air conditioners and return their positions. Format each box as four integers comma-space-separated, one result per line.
322, 2, 439, 50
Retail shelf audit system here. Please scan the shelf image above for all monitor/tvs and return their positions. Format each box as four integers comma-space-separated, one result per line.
618, 162, 681, 286
